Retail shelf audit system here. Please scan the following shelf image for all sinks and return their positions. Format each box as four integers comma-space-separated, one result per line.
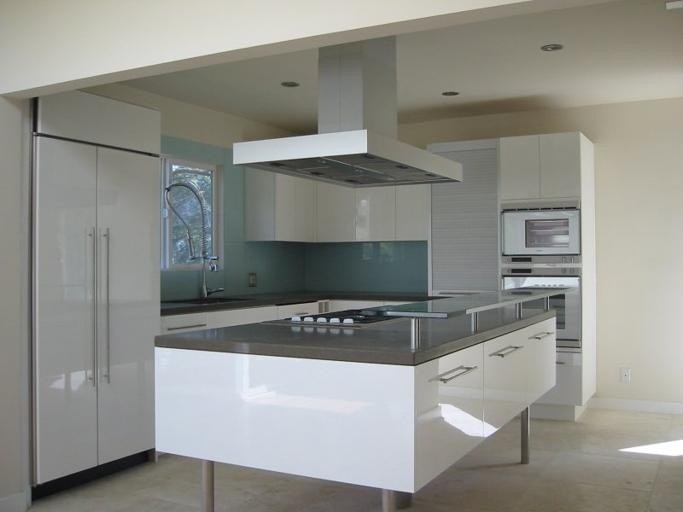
160, 295, 256, 305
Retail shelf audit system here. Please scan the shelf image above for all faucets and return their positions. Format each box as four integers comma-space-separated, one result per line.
165, 182, 225, 299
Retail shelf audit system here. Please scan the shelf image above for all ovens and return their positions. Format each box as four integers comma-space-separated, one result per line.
498, 265, 580, 350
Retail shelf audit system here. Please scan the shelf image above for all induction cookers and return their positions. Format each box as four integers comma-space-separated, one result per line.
283, 307, 396, 326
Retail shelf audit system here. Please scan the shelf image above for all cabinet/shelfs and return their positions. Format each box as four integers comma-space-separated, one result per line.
485, 319, 526, 439
356, 182, 426, 242
316, 181, 355, 244
525, 311, 557, 408
243, 167, 315, 242
500, 133, 597, 204
415, 342, 485, 494
161, 298, 409, 334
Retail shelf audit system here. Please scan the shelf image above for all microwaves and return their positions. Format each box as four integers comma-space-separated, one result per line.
498, 208, 582, 257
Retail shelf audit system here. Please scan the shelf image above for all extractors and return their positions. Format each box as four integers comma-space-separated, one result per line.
233, 38, 465, 190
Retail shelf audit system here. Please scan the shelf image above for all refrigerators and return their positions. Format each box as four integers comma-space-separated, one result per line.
27, 87, 166, 489
